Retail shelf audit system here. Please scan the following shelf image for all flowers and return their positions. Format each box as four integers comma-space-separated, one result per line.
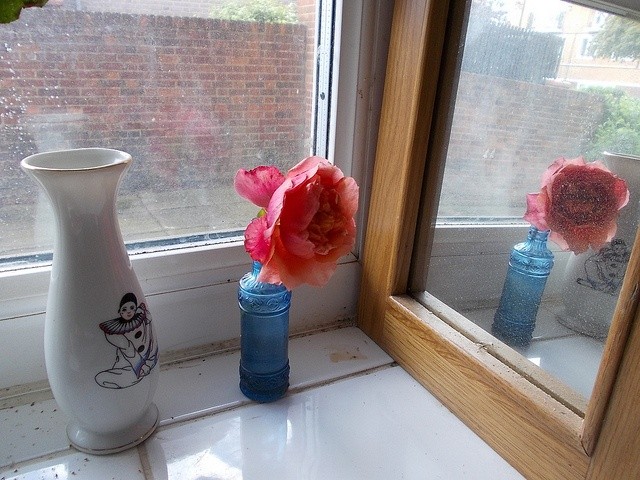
234, 156, 360, 293
526, 156, 631, 256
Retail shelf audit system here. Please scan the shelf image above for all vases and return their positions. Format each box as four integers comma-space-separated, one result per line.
238, 259, 292, 404
493, 225, 554, 345
554, 151, 640, 338
21, 148, 159, 455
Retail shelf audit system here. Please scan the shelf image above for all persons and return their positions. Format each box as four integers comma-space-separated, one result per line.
93, 292, 160, 389
576, 238, 631, 298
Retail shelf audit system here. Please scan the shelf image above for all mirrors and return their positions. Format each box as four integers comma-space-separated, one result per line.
353, 0, 640, 480
